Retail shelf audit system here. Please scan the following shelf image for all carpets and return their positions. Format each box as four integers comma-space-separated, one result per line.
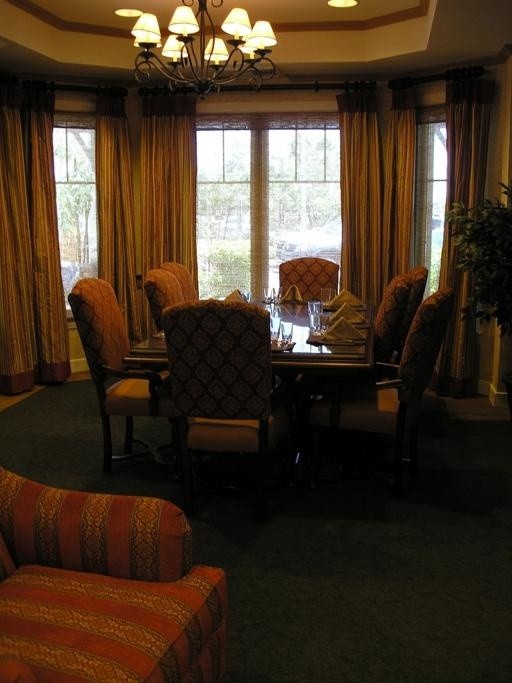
0, 379, 512, 683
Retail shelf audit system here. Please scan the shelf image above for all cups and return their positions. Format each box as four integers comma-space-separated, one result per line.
307, 287, 331, 336
264, 287, 282, 304
281, 321, 294, 340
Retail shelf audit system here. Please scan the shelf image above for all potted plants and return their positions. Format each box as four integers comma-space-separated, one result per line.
446, 183, 512, 421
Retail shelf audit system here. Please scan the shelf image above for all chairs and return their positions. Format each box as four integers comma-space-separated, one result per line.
160, 301, 297, 523
69, 277, 189, 482
305, 288, 453, 488
0, 469, 227, 683
147, 257, 428, 378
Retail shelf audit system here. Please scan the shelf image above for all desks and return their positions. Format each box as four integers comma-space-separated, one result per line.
125, 300, 375, 467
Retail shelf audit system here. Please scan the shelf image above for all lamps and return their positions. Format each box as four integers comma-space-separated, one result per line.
132, 0, 277, 99
